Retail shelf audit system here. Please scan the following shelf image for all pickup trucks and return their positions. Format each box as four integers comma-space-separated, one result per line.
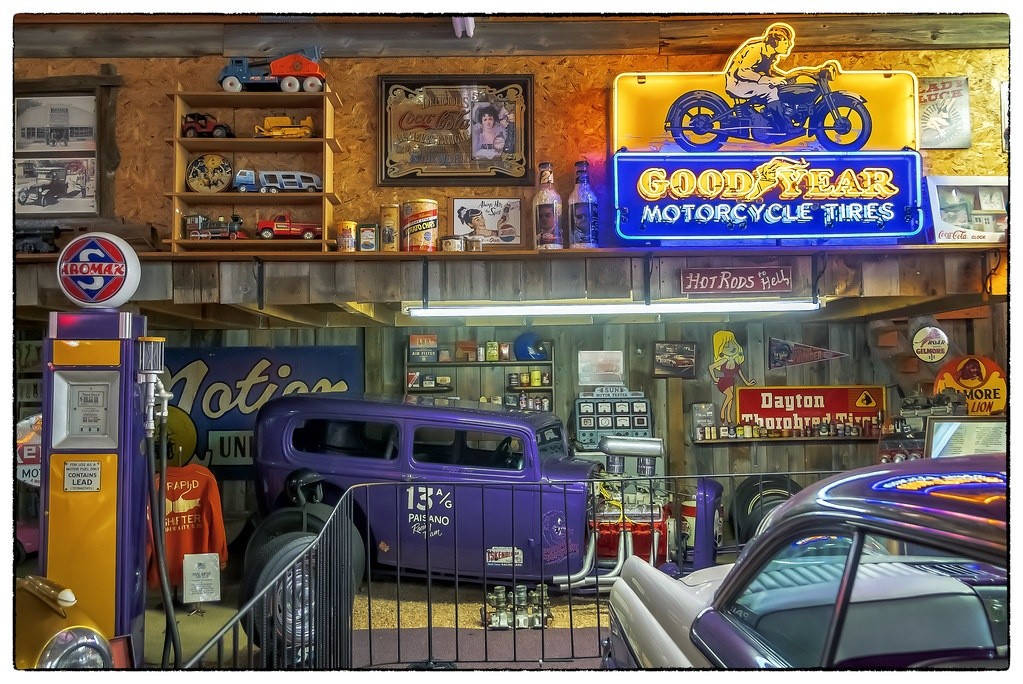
256, 213, 322, 240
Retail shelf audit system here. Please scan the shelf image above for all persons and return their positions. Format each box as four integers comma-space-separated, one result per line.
62, 130, 68, 145
571, 202, 597, 244
51, 129, 56, 145
538, 205, 562, 244
76, 173, 87, 198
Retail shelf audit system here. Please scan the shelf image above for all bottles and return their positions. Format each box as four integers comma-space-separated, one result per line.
531, 163, 564, 249
569, 161, 600, 248
695, 416, 862, 441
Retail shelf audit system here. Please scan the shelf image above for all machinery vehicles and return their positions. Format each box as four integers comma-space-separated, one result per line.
251, 113, 315, 139
216, 46, 327, 93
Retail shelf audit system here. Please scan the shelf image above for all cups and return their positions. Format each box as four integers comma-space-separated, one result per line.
467, 236, 483, 252
441, 236, 465, 252
864, 419, 872, 435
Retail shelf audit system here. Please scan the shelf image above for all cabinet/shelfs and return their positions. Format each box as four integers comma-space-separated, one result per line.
162, 81, 344, 253
403, 341, 555, 418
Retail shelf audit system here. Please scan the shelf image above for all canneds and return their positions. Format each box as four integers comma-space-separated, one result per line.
508, 370, 541, 386
440, 235, 467, 253
402, 199, 439, 251
336, 221, 357, 252
467, 239, 483, 251
477, 347, 485, 361
528, 397, 550, 411
380, 204, 401, 253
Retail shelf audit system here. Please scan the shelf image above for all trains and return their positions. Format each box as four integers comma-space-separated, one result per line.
184, 213, 251, 240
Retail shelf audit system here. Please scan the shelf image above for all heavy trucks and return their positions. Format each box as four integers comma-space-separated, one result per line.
234, 169, 322, 193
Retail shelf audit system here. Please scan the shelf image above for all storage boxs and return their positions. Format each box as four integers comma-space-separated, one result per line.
409, 334, 438, 348
409, 348, 438, 363
920, 176, 1010, 245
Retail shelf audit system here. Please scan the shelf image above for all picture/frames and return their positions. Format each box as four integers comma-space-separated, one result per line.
447, 196, 526, 249
651, 341, 699, 379
376, 73, 535, 188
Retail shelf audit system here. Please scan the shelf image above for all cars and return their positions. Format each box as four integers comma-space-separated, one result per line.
184, 112, 229, 138
598, 453, 1008, 669
18, 167, 68, 209
243, 392, 725, 595
